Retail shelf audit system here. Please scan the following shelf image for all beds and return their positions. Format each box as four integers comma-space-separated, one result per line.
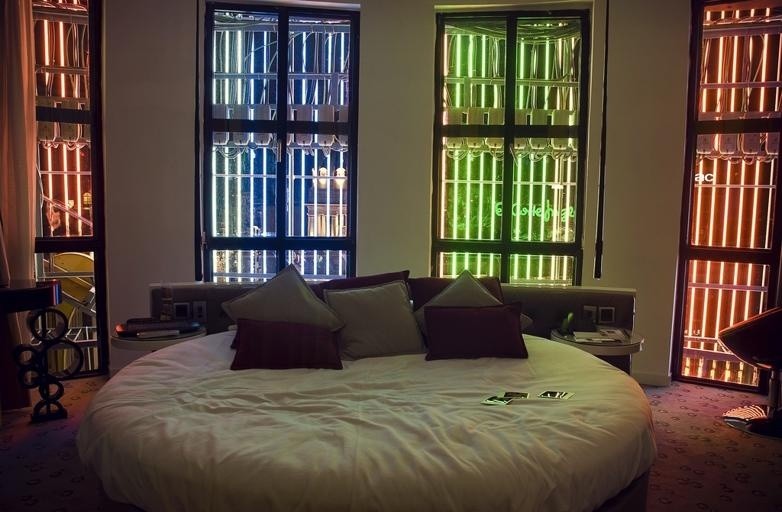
77, 284, 658, 511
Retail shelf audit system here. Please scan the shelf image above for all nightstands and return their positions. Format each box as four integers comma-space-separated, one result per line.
111, 328, 207, 350
551, 326, 645, 356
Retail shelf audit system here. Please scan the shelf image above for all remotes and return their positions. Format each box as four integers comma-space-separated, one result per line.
137, 329, 179, 339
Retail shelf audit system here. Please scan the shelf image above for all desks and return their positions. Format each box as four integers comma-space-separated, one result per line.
0, 279, 84, 424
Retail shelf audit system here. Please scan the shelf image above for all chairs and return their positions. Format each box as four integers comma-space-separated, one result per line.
717, 306, 782, 437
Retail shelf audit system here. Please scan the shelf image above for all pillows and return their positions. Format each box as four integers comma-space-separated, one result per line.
230, 318, 345, 372
413, 270, 532, 335
223, 264, 346, 331
423, 300, 529, 360
323, 281, 425, 360
409, 276, 503, 310
313, 270, 411, 302
